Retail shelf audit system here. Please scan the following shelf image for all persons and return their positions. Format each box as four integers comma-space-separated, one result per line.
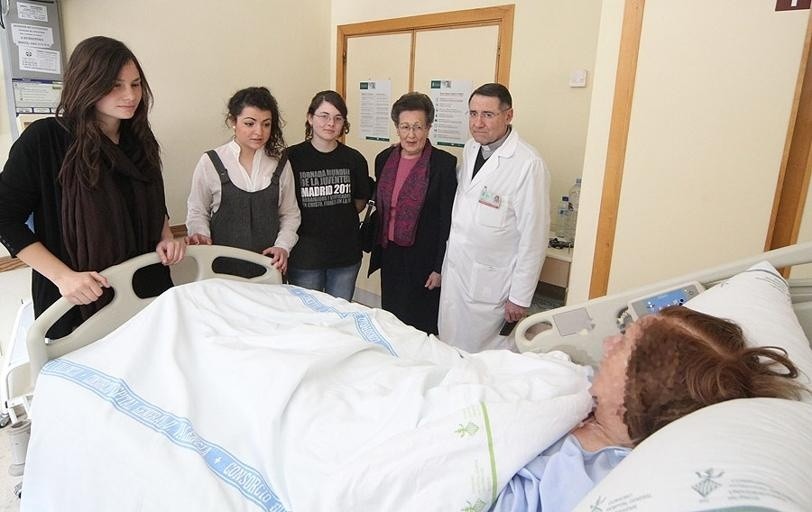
2, 35, 186, 343
489, 304, 811, 511
366, 90, 458, 339
278, 90, 373, 302
436, 84, 553, 355
183, 86, 303, 285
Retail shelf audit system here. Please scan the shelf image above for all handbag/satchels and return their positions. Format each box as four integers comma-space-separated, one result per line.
359, 207, 381, 253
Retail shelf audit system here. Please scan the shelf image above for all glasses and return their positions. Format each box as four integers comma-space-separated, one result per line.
397, 123, 429, 131
462, 108, 512, 120
311, 112, 346, 125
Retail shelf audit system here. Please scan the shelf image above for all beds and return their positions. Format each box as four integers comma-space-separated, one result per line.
21, 241, 812, 512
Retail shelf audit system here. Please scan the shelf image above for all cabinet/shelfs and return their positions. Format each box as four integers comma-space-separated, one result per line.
535, 237, 572, 288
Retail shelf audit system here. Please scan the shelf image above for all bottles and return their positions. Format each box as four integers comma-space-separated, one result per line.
565, 178, 581, 244
556, 197, 568, 239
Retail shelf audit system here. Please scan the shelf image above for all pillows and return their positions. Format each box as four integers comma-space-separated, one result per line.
565, 258, 812, 512
